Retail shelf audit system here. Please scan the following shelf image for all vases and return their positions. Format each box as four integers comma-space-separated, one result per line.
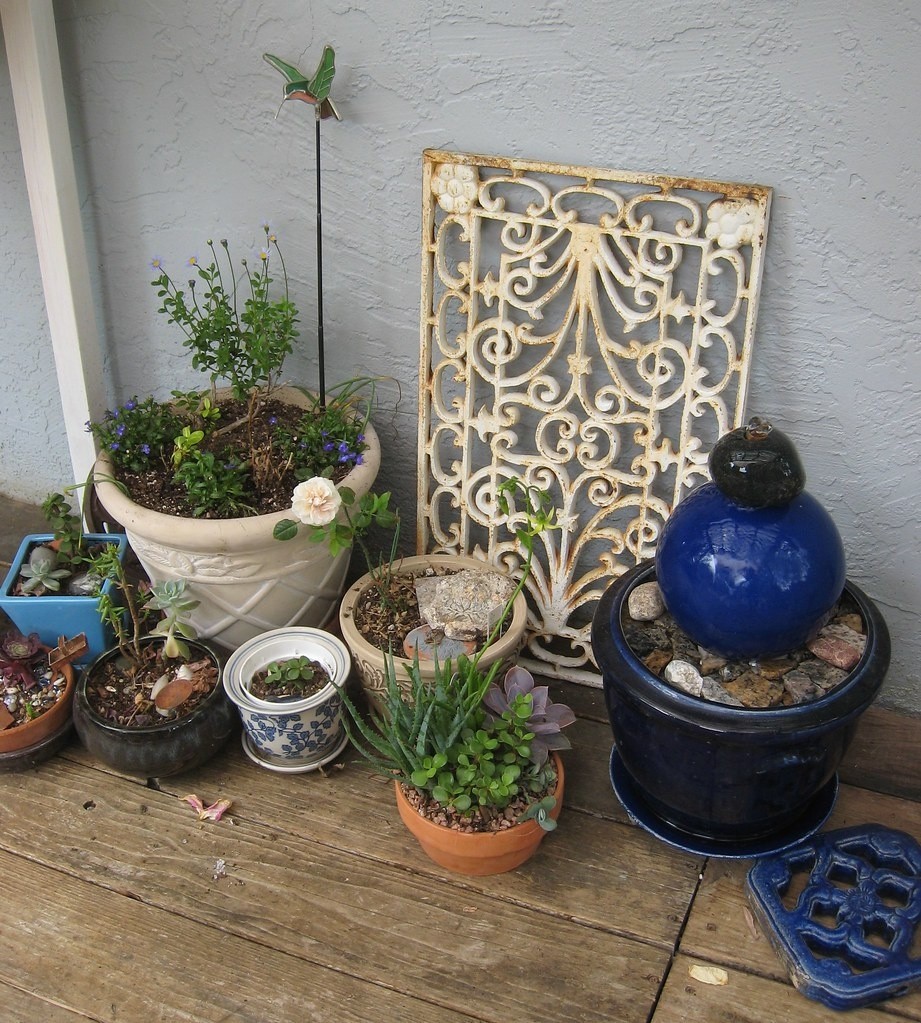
339, 555, 528, 723
94, 386, 380, 652
591, 558, 891, 860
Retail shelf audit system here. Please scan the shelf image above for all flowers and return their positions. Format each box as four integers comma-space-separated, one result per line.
67, 46, 402, 520
272, 476, 401, 614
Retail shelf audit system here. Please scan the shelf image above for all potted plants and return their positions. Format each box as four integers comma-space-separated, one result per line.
0, 605, 89, 771
223, 627, 351, 775
0, 493, 132, 671
322, 475, 579, 874
73, 541, 233, 779
